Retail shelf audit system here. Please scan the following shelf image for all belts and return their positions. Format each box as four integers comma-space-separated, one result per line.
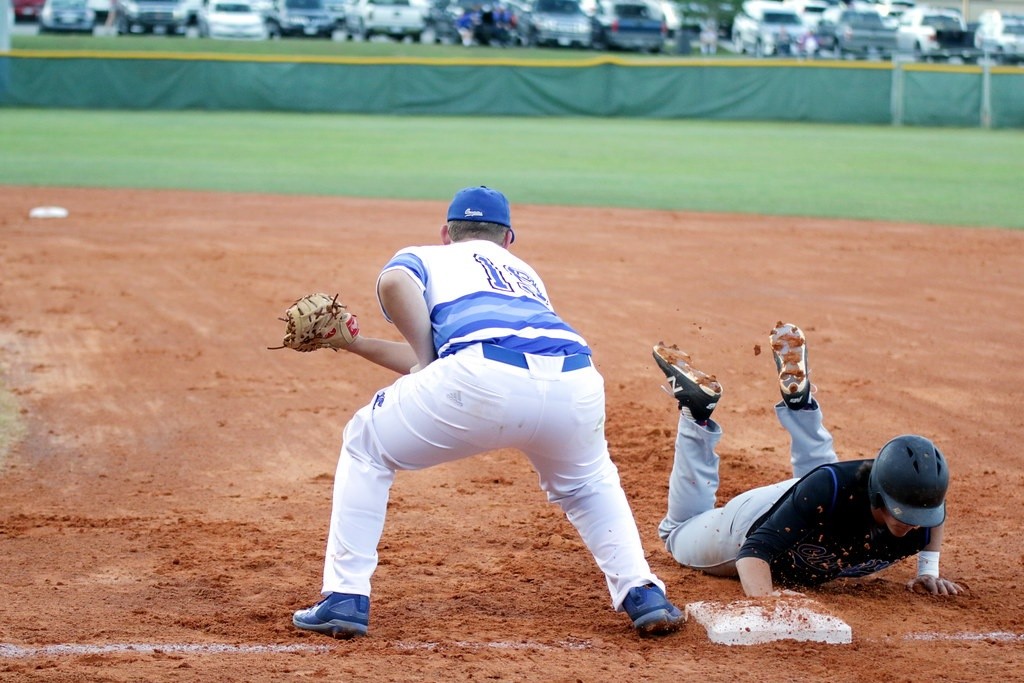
481, 343, 592, 372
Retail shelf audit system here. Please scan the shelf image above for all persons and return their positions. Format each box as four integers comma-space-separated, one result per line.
652, 321, 964, 599
266, 186, 686, 635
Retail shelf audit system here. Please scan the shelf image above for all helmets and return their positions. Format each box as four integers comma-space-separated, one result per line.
870, 434, 950, 528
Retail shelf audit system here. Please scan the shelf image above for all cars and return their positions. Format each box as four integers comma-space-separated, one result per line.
14, 0, 100, 34
343, 0, 674, 55
114, 0, 338, 39
730, 0, 1023, 67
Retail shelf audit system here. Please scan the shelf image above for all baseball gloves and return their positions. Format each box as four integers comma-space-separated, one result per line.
282, 293, 360, 352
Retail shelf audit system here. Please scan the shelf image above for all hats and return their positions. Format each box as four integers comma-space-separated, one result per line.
446, 185, 515, 245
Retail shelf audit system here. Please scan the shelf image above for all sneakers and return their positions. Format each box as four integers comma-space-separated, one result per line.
293, 592, 371, 636
770, 324, 817, 410
621, 585, 684, 636
653, 345, 723, 426
